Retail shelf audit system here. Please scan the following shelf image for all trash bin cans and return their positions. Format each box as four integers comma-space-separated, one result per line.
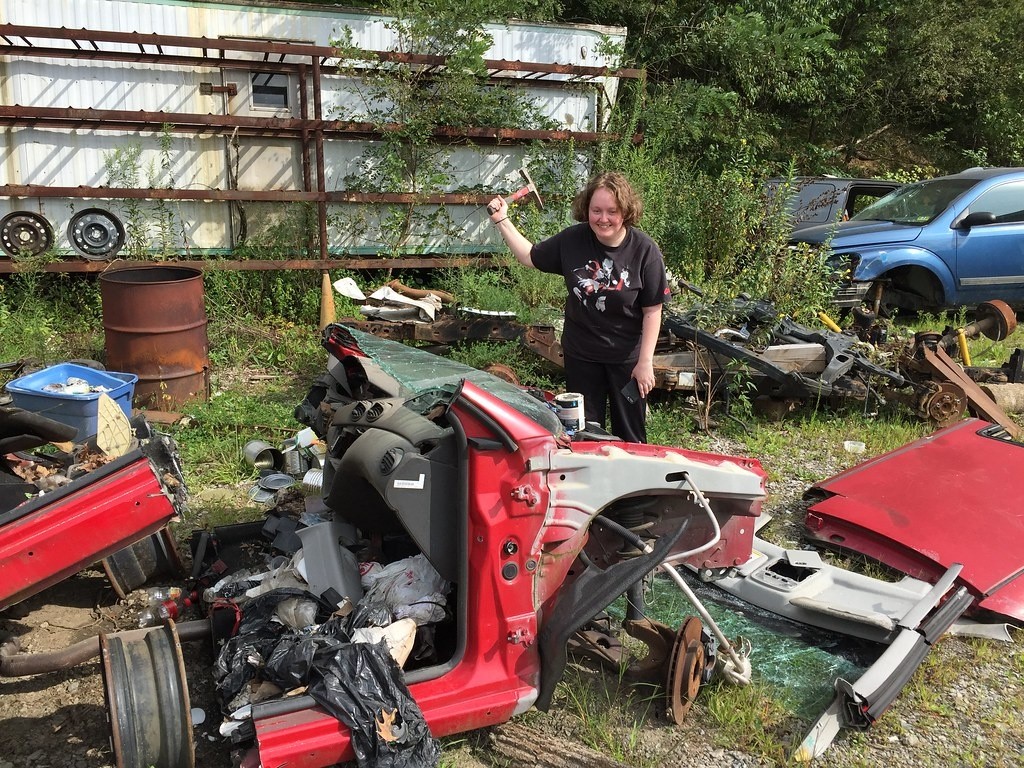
6, 363, 139, 444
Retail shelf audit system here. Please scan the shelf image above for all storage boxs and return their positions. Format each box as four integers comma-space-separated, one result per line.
6, 362, 138, 445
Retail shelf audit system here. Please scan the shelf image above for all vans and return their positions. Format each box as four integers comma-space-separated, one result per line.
760, 177, 912, 240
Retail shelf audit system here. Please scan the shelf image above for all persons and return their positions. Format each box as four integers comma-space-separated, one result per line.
488, 172, 672, 443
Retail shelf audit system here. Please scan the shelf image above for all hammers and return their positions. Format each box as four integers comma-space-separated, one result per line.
486, 167, 545, 216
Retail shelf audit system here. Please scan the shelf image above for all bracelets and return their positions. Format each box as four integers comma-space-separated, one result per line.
495, 217, 509, 225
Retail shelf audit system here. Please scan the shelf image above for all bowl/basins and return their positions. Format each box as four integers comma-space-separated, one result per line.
844, 441, 866, 454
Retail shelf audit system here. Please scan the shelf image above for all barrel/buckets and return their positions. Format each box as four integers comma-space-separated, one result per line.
244, 439, 323, 496
554, 392, 586, 432
99, 264, 211, 413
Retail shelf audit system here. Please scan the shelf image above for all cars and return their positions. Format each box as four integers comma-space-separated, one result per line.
777, 166, 1024, 318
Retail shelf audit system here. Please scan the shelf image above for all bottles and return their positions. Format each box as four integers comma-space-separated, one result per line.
147, 586, 197, 607
136, 598, 192, 628
